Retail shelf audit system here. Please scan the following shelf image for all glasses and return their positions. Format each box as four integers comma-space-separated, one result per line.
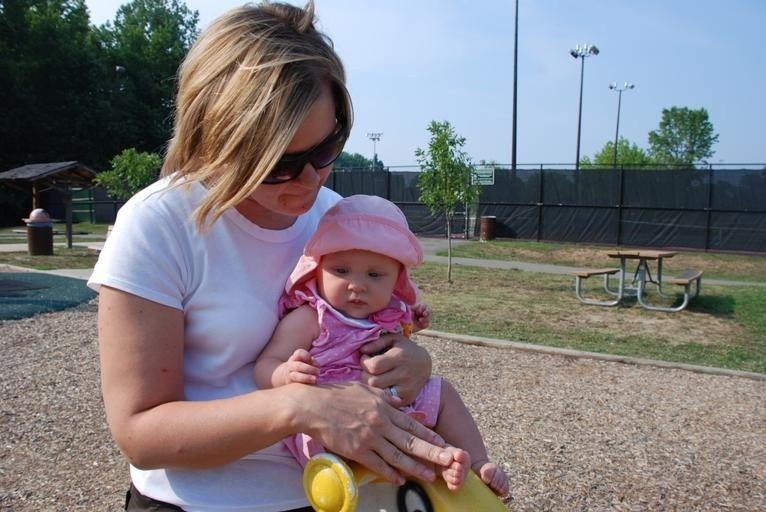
261, 112, 347, 184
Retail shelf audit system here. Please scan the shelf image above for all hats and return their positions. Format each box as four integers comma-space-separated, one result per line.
284, 194, 422, 306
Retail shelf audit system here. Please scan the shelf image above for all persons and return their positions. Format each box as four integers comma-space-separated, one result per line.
249, 190, 511, 498
84, 0, 518, 512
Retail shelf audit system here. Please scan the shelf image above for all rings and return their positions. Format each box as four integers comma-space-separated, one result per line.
390, 386, 399, 396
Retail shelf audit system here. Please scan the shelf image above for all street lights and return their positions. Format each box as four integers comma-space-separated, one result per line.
567, 43, 599, 171
366, 130, 382, 171
609, 81, 634, 168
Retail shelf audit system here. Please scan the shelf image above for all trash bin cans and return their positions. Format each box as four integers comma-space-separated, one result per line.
480, 216, 496, 241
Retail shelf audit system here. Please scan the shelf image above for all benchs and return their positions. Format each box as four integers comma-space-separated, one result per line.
571, 268, 703, 312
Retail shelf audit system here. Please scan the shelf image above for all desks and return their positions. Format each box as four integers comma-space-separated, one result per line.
606, 250, 678, 311
